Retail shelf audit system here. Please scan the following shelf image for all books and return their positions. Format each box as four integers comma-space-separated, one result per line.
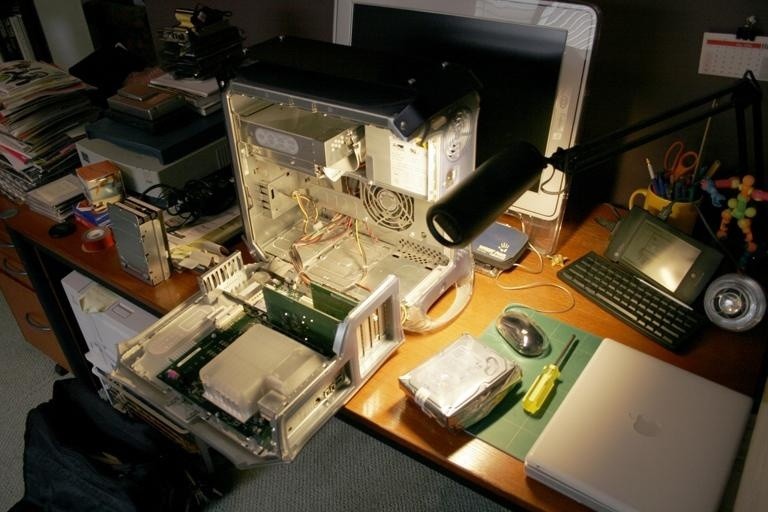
0, 59, 98, 224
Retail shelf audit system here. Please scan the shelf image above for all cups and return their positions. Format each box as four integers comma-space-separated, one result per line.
628, 181, 704, 237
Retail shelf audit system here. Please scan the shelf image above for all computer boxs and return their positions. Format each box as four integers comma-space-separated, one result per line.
109, 33, 483, 471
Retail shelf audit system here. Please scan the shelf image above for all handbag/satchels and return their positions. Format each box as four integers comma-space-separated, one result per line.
7, 378, 236, 512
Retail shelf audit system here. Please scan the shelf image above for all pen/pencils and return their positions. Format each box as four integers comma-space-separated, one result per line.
643, 155, 722, 205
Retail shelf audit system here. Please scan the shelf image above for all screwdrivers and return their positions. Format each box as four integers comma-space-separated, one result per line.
522, 334, 576, 414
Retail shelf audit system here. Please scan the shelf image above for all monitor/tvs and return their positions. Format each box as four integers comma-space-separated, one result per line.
333, 1, 601, 271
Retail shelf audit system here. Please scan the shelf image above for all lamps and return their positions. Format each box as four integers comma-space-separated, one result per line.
427, 68, 767, 250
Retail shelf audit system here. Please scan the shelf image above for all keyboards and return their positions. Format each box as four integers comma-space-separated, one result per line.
556, 250, 706, 355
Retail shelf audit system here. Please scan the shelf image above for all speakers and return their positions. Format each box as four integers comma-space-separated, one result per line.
705, 274, 766, 334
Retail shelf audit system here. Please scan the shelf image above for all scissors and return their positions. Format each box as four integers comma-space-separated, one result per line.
663, 141, 698, 181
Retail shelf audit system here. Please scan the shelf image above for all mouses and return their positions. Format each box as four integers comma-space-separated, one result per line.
496, 310, 550, 356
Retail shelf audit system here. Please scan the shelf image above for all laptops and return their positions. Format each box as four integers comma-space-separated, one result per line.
525, 339, 754, 512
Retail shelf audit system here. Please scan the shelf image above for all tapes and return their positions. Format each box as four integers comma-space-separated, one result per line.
82, 226, 112, 251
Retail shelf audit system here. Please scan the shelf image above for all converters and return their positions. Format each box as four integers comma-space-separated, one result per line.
186, 163, 238, 217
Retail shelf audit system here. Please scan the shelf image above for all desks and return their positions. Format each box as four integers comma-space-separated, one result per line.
0, 192, 767, 511
